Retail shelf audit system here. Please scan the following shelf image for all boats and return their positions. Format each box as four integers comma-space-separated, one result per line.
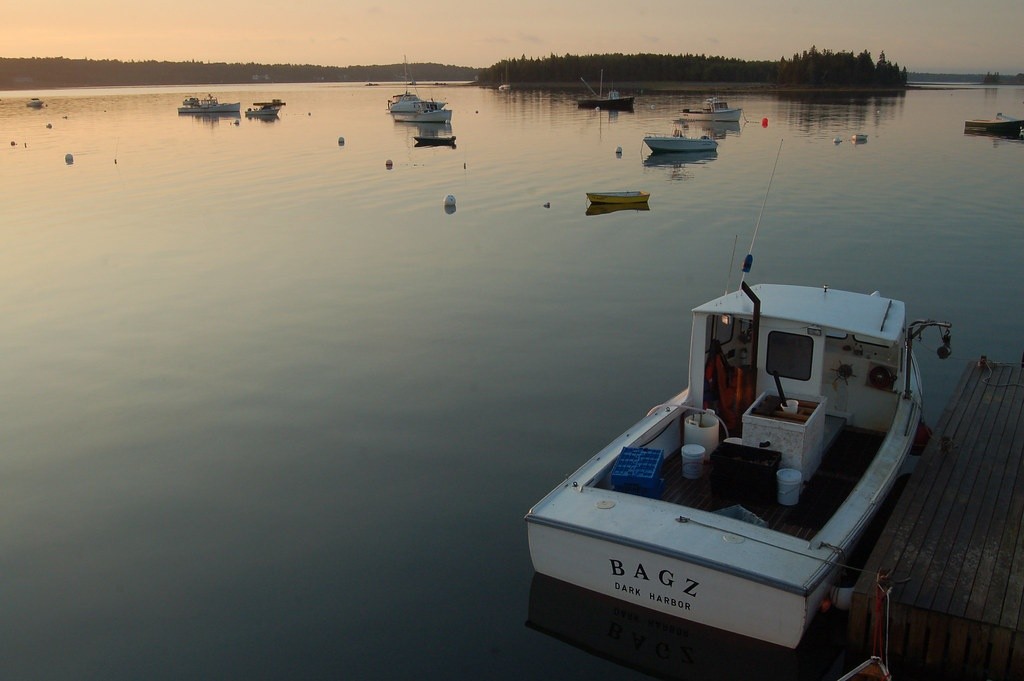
413, 136, 456, 143
244, 105, 280, 115
965, 113, 1024, 139
584, 190, 651, 204
26, 97, 44, 108
643, 151, 718, 168
677, 96, 743, 122
392, 109, 452, 123
523, 136, 953, 652
577, 69, 635, 111
386, 55, 447, 114
642, 134, 718, 152
414, 141, 457, 150
176, 94, 241, 113
585, 203, 650, 216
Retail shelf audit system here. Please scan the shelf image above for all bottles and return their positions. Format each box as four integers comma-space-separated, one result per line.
740, 350, 746, 366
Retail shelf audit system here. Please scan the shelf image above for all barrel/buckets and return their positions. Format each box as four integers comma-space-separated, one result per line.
776, 469, 802, 505
723, 437, 742, 446
684, 413, 719, 461
681, 444, 705, 479
781, 400, 798, 414
